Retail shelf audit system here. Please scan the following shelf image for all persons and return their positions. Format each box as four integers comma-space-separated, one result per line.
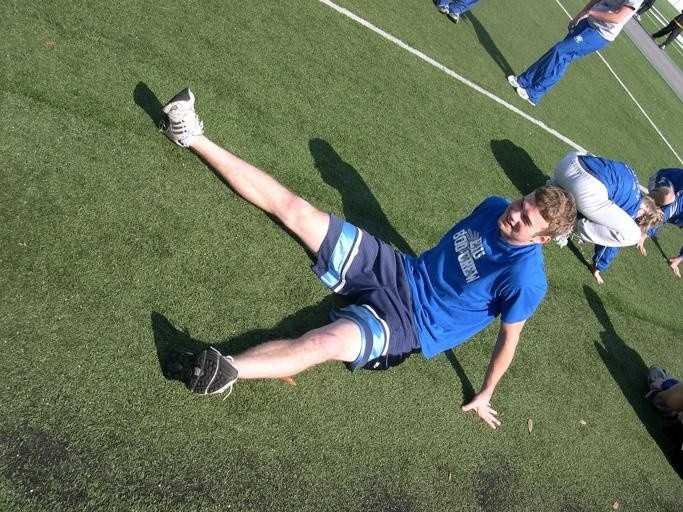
540, 149, 662, 287
639, 165, 683, 277
506, 0, 646, 107
634, 0, 655, 22
434, 0, 483, 24
650, 9, 683, 50
640, 365, 683, 421
149, 83, 578, 432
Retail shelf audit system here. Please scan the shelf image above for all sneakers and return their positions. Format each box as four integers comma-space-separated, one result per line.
645, 365, 683, 421
633, 12, 666, 50
552, 231, 568, 248
517, 87, 537, 106
508, 75, 524, 88
437, 1, 461, 24
162, 345, 240, 397
158, 87, 205, 148
